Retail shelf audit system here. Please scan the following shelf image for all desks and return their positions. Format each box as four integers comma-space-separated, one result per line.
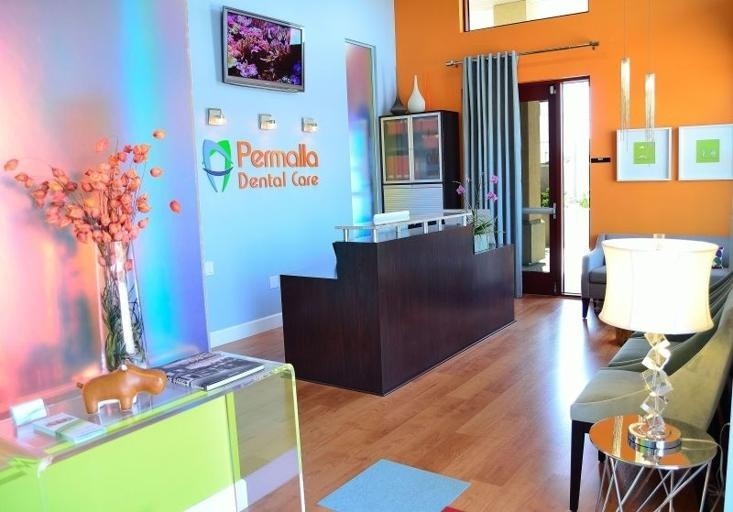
588, 414, 717, 512
0, 350, 308, 511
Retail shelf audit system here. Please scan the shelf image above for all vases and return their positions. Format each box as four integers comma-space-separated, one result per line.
390, 88, 406, 115
473, 233, 489, 252
93, 242, 151, 373
407, 75, 425, 113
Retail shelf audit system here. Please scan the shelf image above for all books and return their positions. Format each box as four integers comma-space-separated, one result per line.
33, 410, 105, 444
150, 350, 265, 392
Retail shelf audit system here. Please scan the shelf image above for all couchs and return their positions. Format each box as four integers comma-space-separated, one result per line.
580, 234, 732, 318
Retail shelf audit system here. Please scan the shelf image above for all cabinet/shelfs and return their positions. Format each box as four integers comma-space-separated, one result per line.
377, 110, 461, 230
276, 210, 519, 398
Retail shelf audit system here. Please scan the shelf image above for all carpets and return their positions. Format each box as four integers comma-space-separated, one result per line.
314, 457, 472, 512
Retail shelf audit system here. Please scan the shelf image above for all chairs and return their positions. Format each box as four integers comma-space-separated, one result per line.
629, 271, 733, 342
569, 292, 732, 511
600, 283, 733, 375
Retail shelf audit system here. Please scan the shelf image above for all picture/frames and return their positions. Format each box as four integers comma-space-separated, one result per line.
220, 5, 306, 94
677, 124, 732, 180
615, 126, 671, 182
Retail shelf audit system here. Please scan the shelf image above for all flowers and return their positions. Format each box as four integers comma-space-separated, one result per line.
0, 128, 183, 279
443, 170, 510, 235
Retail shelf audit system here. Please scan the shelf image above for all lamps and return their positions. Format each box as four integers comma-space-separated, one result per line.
644, 1, 658, 146
619, 0, 633, 144
594, 238, 721, 450
